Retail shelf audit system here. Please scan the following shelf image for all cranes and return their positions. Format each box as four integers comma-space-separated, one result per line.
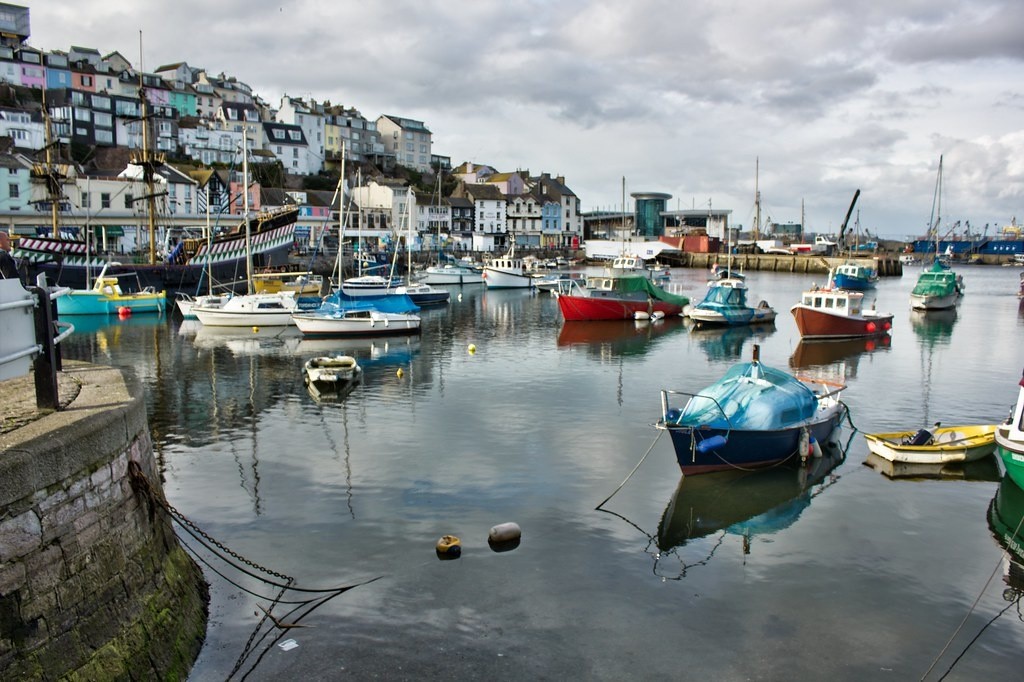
751, 156, 770, 240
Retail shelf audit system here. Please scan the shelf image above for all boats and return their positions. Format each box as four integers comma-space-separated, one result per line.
987, 470, 1024, 563
301, 351, 364, 406
864, 422, 1002, 466
832, 260, 880, 291
648, 342, 850, 476
789, 338, 892, 370
993, 367, 1024, 491
657, 436, 846, 579
789, 281, 895, 339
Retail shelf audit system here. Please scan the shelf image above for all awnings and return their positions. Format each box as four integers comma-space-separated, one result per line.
95, 225, 125, 237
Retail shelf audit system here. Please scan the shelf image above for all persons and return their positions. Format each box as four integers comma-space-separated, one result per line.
0, 231, 21, 280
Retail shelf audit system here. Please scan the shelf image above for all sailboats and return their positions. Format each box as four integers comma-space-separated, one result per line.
908, 154, 966, 310
553, 176, 690, 321
6, 27, 1023, 333
688, 211, 778, 323
